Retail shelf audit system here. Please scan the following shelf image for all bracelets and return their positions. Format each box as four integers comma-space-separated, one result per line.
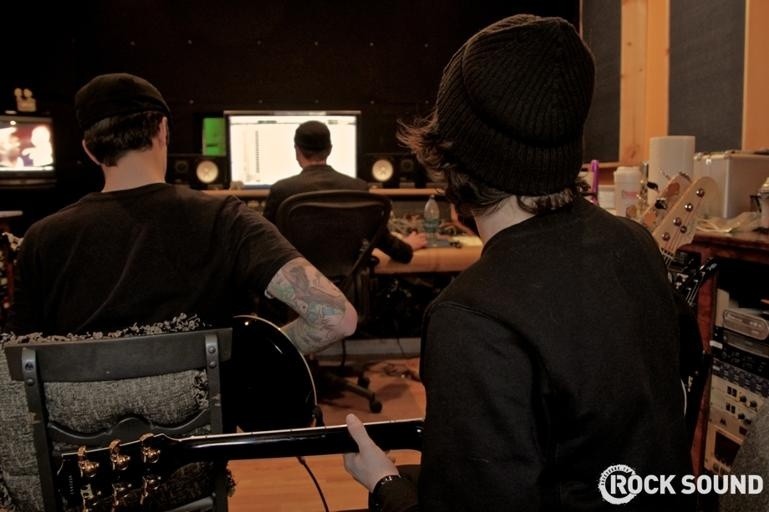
372, 474, 399, 504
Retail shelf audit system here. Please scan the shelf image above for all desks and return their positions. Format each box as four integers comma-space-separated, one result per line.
199, 186, 483, 276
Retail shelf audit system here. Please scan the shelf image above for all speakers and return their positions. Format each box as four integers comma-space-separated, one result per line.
165, 154, 226, 189
366, 152, 428, 189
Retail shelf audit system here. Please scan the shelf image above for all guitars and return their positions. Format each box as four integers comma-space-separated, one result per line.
51, 353, 714, 512
0, 233, 319, 430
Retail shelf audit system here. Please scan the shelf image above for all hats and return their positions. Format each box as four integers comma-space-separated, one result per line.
432, 14, 594, 194
74, 73, 171, 134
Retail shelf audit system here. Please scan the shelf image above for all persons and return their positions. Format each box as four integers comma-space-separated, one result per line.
1, 72, 360, 437
264, 120, 430, 363
0, 124, 33, 169
340, 10, 702, 512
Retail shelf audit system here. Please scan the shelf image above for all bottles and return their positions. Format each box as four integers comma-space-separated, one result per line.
424, 194, 440, 247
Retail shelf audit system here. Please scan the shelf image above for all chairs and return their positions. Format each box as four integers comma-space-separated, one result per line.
275, 190, 393, 414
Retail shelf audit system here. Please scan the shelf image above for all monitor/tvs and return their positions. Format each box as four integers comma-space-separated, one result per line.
0, 115, 58, 185
222, 110, 363, 191
